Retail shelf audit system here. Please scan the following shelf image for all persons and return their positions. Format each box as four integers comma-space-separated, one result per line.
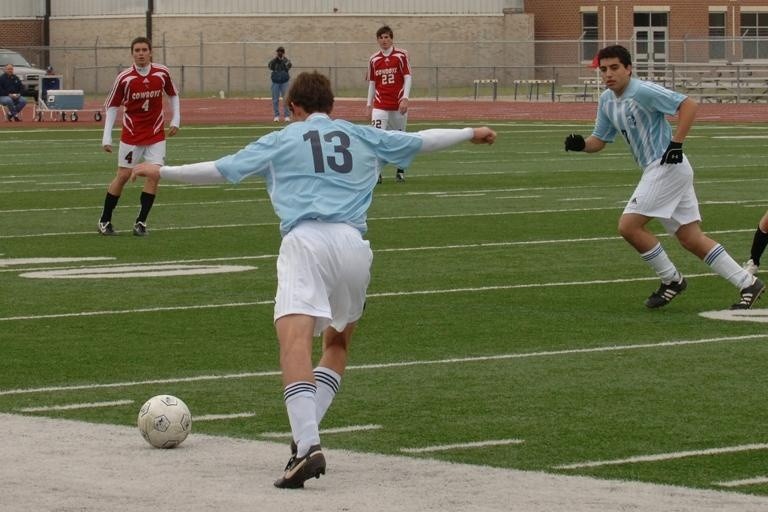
131, 68, 497, 488
268, 47, 292, 122
564, 44, 767, 310
741, 209, 768, 275
44, 67, 55, 75
98, 36, 181, 237
366, 26, 413, 183
0, 64, 26, 122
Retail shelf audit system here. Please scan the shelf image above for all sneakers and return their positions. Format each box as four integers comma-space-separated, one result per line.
396, 173, 405, 184
729, 276, 766, 310
274, 444, 326, 488
284, 116, 290, 122
96, 218, 118, 236
274, 115, 279, 122
14, 116, 22, 121
133, 221, 149, 236
7, 113, 13, 122
743, 259, 757, 274
643, 272, 688, 310
377, 173, 381, 184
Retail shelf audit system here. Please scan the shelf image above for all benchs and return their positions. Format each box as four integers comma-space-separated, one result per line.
546, 67, 768, 104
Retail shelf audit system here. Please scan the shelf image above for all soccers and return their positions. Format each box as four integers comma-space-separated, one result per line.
138, 395, 191, 448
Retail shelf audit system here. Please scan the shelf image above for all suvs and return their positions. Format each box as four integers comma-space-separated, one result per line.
0, 47, 49, 101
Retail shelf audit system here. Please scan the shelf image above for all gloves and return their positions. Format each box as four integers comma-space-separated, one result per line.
660, 141, 682, 165
564, 135, 585, 151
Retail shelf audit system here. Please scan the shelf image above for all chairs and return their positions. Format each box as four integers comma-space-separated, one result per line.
1, 105, 18, 121
